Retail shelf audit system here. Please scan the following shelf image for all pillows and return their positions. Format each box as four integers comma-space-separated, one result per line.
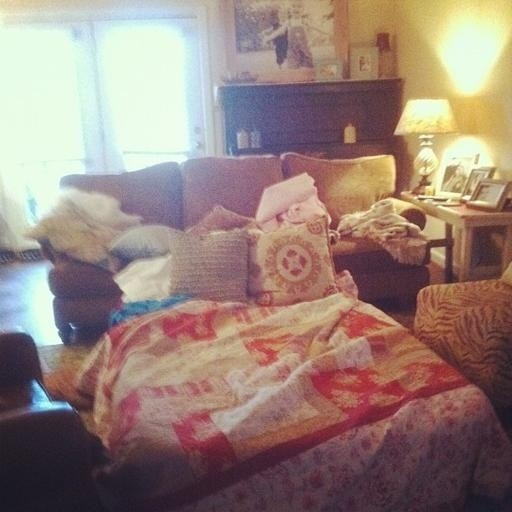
186, 205, 254, 234
108, 225, 184, 261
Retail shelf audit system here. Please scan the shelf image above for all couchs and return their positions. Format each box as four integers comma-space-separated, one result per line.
250, 217, 335, 304
41, 154, 432, 344
416, 261, 511, 423
170, 232, 247, 304
0, 327, 98, 512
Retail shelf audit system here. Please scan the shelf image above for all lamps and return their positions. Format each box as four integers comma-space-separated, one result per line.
395, 99, 456, 195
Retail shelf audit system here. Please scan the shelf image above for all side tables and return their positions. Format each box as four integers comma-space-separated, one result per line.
401, 190, 512, 282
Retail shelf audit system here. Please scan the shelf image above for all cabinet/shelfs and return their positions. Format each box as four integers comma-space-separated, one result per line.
217, 80, 416, 193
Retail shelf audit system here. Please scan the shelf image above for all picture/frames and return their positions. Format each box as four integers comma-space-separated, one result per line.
458, 167, 494, 201
467, 179, 510, 211
439, 153, 478, 200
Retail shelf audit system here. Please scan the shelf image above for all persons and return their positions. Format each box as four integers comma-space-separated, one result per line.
442, 164, 465, 193
257, 7, 288, 70
264, 5, 330, 79
443, 156, 460, 183
